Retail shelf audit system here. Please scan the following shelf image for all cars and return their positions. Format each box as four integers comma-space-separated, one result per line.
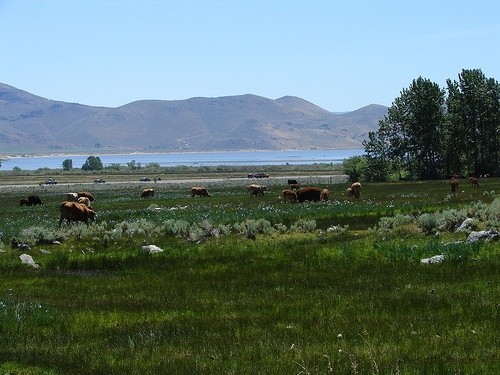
94, 179, 106, 184
45, 178, 57, 184
139, 177, 150, 181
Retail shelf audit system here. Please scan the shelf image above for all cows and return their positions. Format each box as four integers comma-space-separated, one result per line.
77, 192, 96, 203
249, 185, 268, 196
352, 183, 362, 198
140, 188, 155, 199
191, 187, 211, 198
28, 194, 43, 207
296, 188, 322, 203
58, 202, 96, 227
67, 193, 79, 202
20, 199, 28, 206
468, 177, 474, 187
451, 177, 459, 192
287, 180, 298, 186
77, 197, 93, 210
321, 189, 330, 201
291, 185, 300, 191
471, 178, 480, 187
282, 190, 297, 203
347, 188, 353, 198
